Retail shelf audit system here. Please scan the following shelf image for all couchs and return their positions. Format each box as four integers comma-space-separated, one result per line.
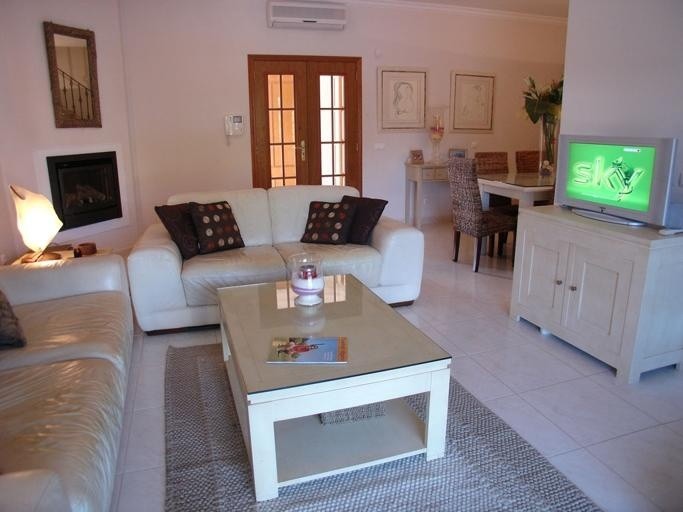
0, 255, 133, 512
127, 185, 424, 333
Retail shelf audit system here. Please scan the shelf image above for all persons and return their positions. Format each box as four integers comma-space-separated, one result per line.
277, 341, 318, 357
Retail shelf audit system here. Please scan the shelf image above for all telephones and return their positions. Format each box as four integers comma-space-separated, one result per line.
224, 114, 243, 136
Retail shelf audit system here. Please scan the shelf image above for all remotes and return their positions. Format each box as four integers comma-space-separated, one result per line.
659, 229, 683, 236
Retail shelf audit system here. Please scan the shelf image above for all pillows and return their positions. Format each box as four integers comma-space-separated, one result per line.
154, 183, 388, 259
0, 292, 27, 349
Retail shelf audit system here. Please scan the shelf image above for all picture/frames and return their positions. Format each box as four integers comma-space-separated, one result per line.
449, 72, 496, 133
378, 65, 425, 129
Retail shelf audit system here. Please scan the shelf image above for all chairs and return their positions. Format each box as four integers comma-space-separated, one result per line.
447, 149, 557, 272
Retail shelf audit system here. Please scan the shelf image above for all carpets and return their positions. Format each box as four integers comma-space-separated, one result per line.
165, 342, 603, 512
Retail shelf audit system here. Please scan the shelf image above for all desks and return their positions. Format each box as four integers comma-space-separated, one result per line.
405, 164, 447, 229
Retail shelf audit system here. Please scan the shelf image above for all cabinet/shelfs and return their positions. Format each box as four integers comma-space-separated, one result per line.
510, 204, 683, 383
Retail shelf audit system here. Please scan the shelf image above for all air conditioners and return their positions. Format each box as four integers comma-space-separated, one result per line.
267, 1, 347, 31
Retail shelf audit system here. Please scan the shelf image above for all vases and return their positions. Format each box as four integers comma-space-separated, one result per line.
431, 111, 445, 162
539, 120, 557, 177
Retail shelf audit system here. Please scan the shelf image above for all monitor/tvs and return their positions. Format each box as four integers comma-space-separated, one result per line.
553, 136, 683, 229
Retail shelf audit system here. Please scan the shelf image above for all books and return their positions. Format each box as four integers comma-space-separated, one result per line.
264, 336, 349, 364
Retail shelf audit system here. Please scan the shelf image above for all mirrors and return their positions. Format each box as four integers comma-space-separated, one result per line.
44, 18, 103, 130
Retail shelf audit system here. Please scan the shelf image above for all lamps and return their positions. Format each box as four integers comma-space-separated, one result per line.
10, 186, 63, 262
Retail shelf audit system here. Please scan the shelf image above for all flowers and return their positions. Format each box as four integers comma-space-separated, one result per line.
520, 74, 559, 165
431, 127, 444, 141
